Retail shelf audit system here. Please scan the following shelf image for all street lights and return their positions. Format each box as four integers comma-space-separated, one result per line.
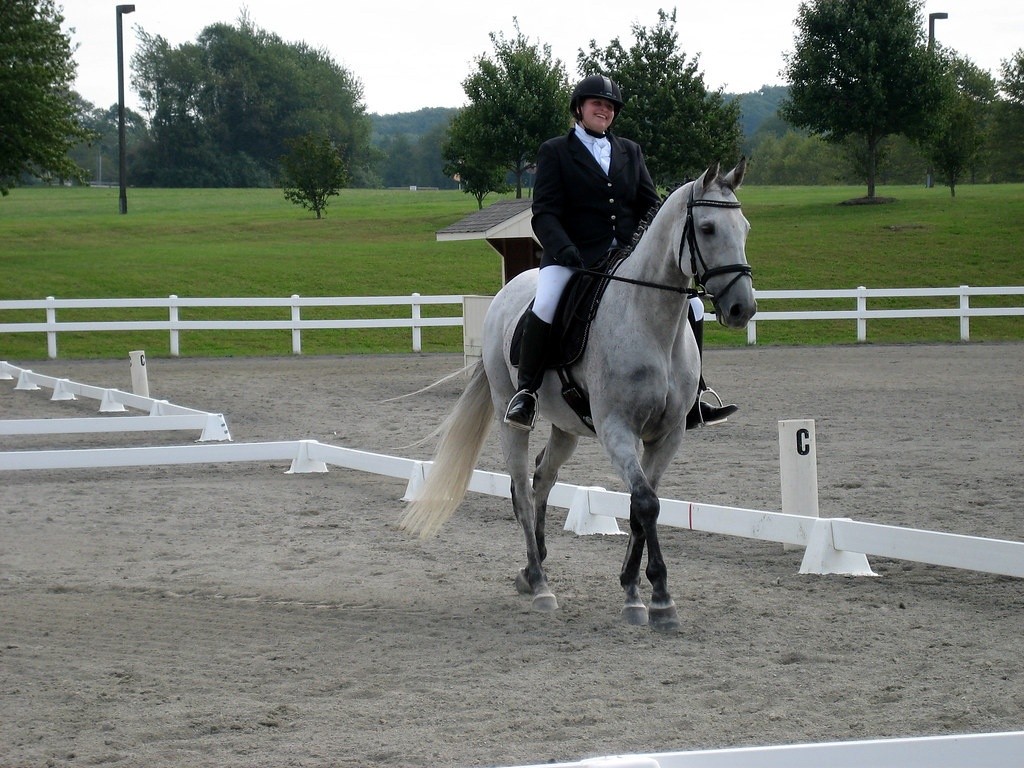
116, 3, 135, 215
927, 12, 947, 188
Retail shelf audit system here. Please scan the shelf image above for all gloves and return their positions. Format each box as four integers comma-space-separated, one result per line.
559, 246, 582, 268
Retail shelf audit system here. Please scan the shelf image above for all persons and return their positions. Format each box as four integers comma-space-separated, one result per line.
505, 75, 740, 431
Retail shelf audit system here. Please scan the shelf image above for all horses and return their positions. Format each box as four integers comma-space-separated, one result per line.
378, 154, 758, 638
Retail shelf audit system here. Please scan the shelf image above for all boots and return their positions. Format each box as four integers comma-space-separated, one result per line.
685, 317, 738, 432
507, 306, 553, 425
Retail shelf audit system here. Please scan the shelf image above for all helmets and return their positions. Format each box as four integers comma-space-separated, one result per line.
569, 75, 625, 119
